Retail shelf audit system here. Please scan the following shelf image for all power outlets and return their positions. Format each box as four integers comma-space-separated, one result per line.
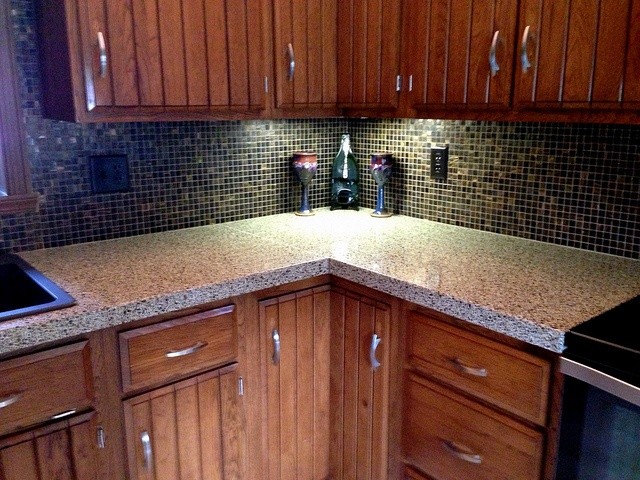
430, 148, 448, 181
90, 155, 130, 195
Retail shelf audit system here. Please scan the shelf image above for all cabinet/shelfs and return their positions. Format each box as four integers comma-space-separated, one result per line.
338, 0, 403, 113
407, 0, 640, 119
0, 408, 102, 477
399, 301, 554, 479
253, 274, 329, 480
274, 0, 337, 118
125, 362, 240, 480
117, 299, 245, 397
328, 274, 391, 479
78, 0, 269, 113
0, 330, 111, 439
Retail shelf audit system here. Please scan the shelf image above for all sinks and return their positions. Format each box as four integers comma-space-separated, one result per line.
0, 254, 77, 322
